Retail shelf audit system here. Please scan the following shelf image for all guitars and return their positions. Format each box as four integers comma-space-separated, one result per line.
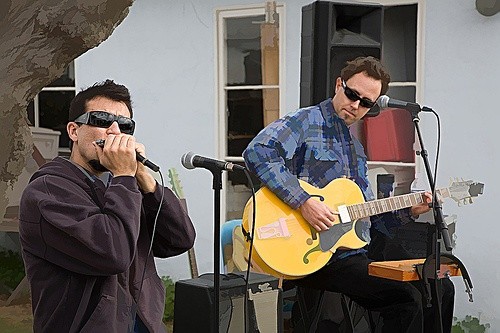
241, 177, 485, 279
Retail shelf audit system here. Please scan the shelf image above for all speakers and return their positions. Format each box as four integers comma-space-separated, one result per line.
299, 0, 385, 117
173, 271, 284, 333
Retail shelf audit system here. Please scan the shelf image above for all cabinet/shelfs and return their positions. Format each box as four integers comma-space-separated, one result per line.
299, 0, 425, 199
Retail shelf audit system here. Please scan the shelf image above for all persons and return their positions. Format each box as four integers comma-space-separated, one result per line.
19, 79, 196, 333
243, 57, 456, 333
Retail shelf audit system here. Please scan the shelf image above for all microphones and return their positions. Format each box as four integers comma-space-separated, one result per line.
136, 150, 160, 172
181, 151, 246, 173
376, 95, 433, 112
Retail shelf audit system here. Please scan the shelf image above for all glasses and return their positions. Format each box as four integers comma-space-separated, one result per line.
74, 111, 135, 136
341, 80, 376, 109
